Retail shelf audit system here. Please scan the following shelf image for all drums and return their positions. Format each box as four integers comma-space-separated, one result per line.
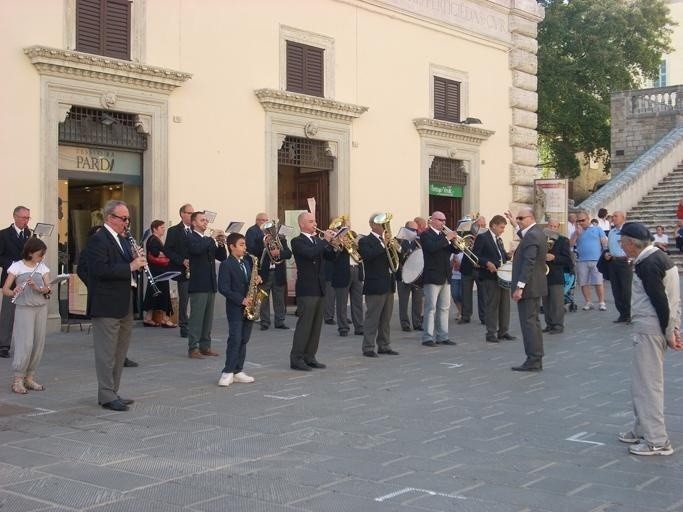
401, 248, 425, 287
496, 260, 513, 290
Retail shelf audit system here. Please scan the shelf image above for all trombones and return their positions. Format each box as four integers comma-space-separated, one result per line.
441, 225, 479, 266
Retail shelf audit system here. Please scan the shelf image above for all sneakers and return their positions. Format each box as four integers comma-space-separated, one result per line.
217, 371, 234, 387
122, 357, 139, 368
598, 303, 608, 311
615, 430, 645, 444
232, 371, 255, 383
627, 437, 674, 456
582, 302, 595, 311
188, 349, 207, 359
200, 348, 217, 356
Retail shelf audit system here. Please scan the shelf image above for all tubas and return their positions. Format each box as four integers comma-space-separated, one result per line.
260, 219, 286, 265
373, 211, 400, 272
328, 215, 363, 263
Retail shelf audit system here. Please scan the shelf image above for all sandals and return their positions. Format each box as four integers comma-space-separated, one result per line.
24, 379, 46, 392
11, 378, 29, 394
160, 317, 178, 329
142, 318, 160, 327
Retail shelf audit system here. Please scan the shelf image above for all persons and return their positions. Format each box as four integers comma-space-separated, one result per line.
0, 205, 39, 360
2, 237, 52, 396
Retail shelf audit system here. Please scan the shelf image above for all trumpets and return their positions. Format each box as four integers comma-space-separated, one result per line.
313, 226, 353, 254
205, 226, 231, 246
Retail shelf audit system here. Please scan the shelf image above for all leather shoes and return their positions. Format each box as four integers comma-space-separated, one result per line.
542, 325, 552, 333
402, 325, 425, 332
510, 360, 544, 372
259, 324, 291, 330
377, 349, 400, 355
548, 327, 564, 334
0, 350, 10, 359
324, 317, 364, 337
498, 332, 517, 341
485, 335, 498, 343
435, 338, 456, 346
289, 361, 313, 371
97, 395, 135, 405
362, 350, 377, 358
421, 340, 441, 347
101, 400, 130, 413
452, 312, 484, 325
307, 362, 326, 369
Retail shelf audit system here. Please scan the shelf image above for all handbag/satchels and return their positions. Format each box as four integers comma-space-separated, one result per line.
146, 248, 171, 267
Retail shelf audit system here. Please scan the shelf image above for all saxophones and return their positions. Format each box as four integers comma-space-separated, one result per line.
243, 251, 269, 321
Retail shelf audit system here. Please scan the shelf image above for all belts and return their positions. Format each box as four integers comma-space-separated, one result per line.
610, 257, 628, 261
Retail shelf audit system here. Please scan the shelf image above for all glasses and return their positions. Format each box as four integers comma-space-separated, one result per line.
516, 216, 532, 221
256, 217, 269, 220
432, 216, 445, 221
184, 210, 194, 213
575, 217, 588, 222
111, 213, 132, 222
15, 214, 32, 218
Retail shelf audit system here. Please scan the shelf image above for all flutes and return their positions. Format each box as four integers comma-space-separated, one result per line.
11, 257, 46, 305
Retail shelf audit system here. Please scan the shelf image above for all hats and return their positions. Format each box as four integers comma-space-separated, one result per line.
615, 221, 655, 242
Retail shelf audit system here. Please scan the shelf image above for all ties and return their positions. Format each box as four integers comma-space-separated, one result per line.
116, 232, 138, 282
186, 227, 192, 237
379, 236, 385, 246
310, 236, 316, 245
18, 230, 24, 240
496, 236, 506, 263
202, 235, 208, 241
438, 232, 445, 242
